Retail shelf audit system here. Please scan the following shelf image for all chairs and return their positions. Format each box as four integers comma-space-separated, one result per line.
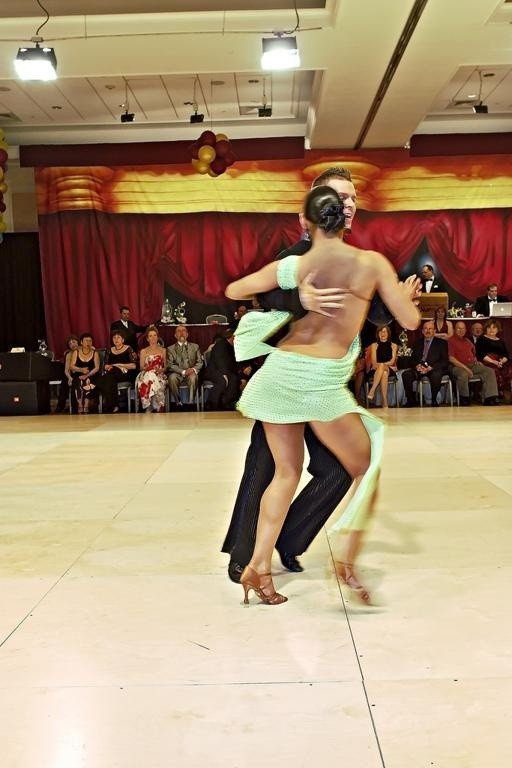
206, 313, 227, 325
200, 343, 214, 411
68, 347, 106, 413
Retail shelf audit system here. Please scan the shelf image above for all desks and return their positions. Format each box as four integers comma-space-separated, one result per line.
391, 317, 512, 353
147, 322, 229, 358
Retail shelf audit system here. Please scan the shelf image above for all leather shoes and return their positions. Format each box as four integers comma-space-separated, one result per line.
276, 539, 305, 574
52, 401, 236, 415
356, 392, 511, 410
227, 558, 248, 582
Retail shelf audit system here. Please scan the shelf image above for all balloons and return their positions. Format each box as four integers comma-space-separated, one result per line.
189, 132, 238, 178
0, 128, 8, 244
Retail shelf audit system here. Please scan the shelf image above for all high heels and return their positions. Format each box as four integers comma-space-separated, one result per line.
323, 558, 382, 611
238, 565, 288, 608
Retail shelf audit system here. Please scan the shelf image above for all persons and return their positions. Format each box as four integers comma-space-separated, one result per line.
349, 265, 510, 409
54, 305, 271, 414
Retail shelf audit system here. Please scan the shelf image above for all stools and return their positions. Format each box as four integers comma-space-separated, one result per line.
364, 374, 400, 407
116, 381, 134, 413
135, 371, 167, 411
457, 377, 485, 407
167, 381, 200, 412
416, 374, 454, 407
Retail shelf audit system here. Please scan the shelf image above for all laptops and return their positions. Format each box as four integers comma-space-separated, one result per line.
490, 303, 512, 318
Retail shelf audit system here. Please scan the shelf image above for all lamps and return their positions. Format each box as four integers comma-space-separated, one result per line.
473, 70, 489, 113
188, 76, 205, 123
259, 0, 304, 71
118, 80, 137, 123
14, 0, 61, 83
258, 77, 272, 117
